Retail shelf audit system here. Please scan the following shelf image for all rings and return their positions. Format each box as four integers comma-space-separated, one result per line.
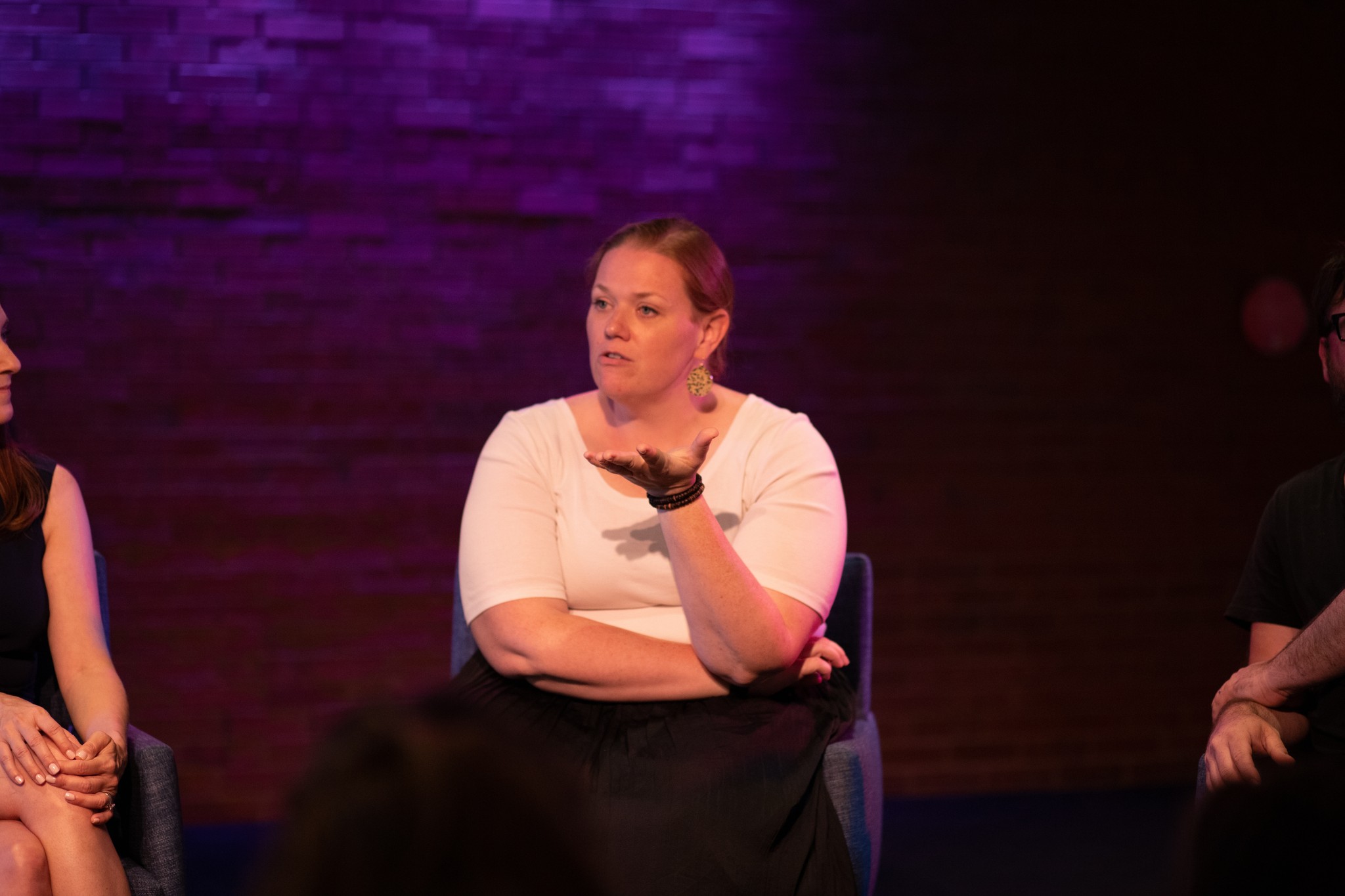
101, 792, 115, 811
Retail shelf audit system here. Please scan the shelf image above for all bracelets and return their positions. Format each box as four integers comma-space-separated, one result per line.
646, 472, 702, 504
649, 483, 704, 510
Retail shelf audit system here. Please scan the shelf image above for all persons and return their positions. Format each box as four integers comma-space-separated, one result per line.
449, 211, 855, 896
273, 681, 598, 895
1201, 252, 1345, 896
0, 305, 133, 896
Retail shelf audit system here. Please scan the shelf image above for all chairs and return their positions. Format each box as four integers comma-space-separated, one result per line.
45, 544, 186, 896
448, 549, 886, 896
